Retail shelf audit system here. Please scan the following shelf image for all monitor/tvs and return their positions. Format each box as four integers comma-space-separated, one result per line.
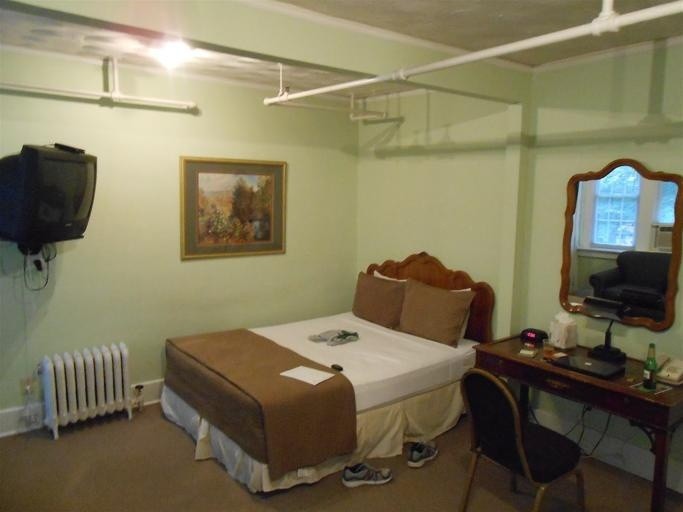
0, 144, 97, 255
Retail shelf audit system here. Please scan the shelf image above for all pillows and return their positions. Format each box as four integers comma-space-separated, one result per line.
349, 265, 477, 349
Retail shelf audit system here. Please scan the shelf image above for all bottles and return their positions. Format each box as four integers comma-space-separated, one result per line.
643, 342, 658, 390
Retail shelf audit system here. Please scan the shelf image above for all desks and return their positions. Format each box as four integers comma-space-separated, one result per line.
469, 332, 683, 512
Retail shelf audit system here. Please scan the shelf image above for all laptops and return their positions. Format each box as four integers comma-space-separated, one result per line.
552, 355, 626, 381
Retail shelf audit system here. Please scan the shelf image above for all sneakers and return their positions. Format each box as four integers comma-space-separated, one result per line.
406, 442, 440, 467
342, 462, 393, 489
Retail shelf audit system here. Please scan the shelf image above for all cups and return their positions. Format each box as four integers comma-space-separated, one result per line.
542, 339, 552, 360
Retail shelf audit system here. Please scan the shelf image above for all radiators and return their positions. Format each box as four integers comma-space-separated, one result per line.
41, 341, 147, 439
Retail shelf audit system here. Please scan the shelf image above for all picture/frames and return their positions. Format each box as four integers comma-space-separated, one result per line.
179, 155, 287, 260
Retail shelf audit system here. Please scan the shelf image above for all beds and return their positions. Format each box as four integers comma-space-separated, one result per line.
165, 250, 497, 499
589, 249, 672, 304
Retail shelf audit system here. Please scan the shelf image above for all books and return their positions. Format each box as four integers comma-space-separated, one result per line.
280, 365, 337, 387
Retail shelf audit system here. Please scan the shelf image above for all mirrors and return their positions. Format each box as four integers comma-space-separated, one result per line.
557, 158, 683, 333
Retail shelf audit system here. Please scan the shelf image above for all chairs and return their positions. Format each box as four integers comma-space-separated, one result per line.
458, 367, 585, 512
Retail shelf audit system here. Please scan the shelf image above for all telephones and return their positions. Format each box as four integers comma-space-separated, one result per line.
655, 354, 683, 385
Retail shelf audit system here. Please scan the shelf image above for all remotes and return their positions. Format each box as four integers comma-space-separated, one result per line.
54, 143, 84, 153
